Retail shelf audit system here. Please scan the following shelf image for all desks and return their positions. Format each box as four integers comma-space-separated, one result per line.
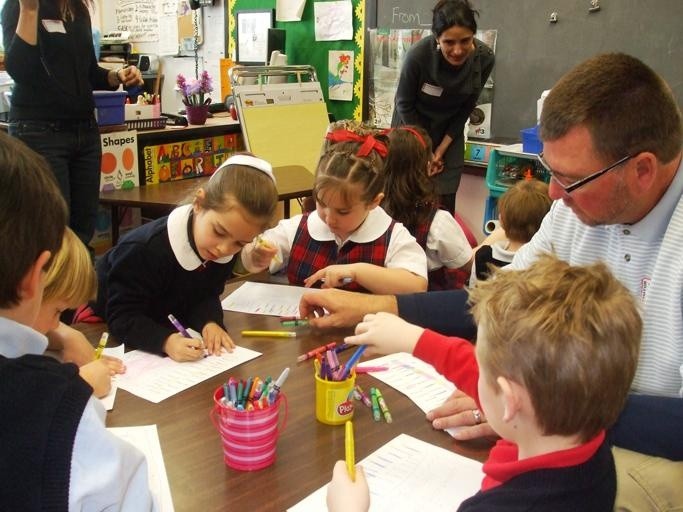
99, 165, 315, 246
44, 279, 682, 510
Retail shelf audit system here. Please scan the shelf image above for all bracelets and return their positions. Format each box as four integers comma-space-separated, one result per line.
114, 69, 120, 87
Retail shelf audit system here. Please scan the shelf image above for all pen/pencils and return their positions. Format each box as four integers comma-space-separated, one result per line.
355, 367, 388, 373
332, 343, 357, 352
339, 369, 350, 381
369, 386, 382, 421
296, 341, 337, 364
355, 383, 372, 408
281, 320, 309, 326
223, 367, 290, 412
279, 316, 308, 321
332, 347, 340, 372
321, 277, 352, 283
313, 358, 321, 377
93, 332, 109, 361
325, 344, 338, 380
256, 235, 280, 263
354, 389, 363, 401
168, 314, 208, 358
325, 364, 333, 381
316, 350, 323, 365
344, 420, 356, 482
375, 387, 393, 424
345, 344, 367, 370
241, 330, 297, 338
320, 356, 326, 380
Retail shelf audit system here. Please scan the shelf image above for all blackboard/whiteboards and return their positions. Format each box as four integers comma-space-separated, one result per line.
368, 1, 683, 145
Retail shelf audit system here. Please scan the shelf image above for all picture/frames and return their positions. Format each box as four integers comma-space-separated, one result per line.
236, 8, 276, 66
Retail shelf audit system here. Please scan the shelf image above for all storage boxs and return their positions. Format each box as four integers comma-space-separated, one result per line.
93, 92, 128, 125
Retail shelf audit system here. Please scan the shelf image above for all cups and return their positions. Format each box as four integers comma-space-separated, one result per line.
315, 370, 357, 426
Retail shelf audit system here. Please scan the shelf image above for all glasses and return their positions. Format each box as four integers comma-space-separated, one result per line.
534, 149, 631, 196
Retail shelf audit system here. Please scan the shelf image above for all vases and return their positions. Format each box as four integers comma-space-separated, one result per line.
185, 106, 208, 125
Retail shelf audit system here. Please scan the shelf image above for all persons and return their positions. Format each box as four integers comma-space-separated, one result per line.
1, 133, 152, 511
1, 0, 144, 242
299, 53, 682, 460
241, 131, 428, 296
30, 226, 126, 398
391, 1, 496, 216
468, 179, 552, 285
74, 153, 278, 363
382, 126, 474, 291
327, 251, 643, 511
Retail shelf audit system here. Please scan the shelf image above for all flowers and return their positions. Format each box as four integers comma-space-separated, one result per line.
176, 70, 213, 106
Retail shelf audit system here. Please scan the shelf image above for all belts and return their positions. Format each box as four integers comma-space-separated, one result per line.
11, 117, 78, 131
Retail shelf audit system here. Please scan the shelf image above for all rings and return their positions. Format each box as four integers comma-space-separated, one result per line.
474, 409, 481, 422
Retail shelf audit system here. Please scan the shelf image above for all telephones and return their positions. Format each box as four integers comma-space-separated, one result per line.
189, 0, 215, 10
